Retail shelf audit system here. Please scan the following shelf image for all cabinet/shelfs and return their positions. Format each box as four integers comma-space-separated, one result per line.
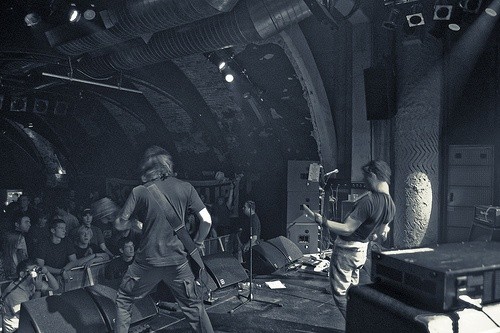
443, 145, 500, 244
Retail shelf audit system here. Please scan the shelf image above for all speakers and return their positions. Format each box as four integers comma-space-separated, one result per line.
467, 221, 500, 242
196, 252, 249, 293
18, 276, 159, 333
249, 160, 320, 275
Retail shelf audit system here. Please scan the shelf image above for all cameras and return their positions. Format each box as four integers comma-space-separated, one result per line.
34, 266, 43, 272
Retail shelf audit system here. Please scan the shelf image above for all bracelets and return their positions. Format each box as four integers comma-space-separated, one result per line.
95, 253, 97, 257
60, 268, 65, 274
322, 217, 327, 227
45, 271, 50, 276
34, 289, 42, 292
193, 239, 206, 247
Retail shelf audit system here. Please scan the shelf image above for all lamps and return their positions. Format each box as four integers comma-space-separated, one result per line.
202, 51, 226, 70
383, 0, 500, 32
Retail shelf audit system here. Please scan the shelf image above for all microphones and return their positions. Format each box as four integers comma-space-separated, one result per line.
325, 169, 339, 176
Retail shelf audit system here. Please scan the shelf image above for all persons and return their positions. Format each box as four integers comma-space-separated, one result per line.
308, 160, 396, 322
112, 144, 217, 333
0, 187, 261, 333
